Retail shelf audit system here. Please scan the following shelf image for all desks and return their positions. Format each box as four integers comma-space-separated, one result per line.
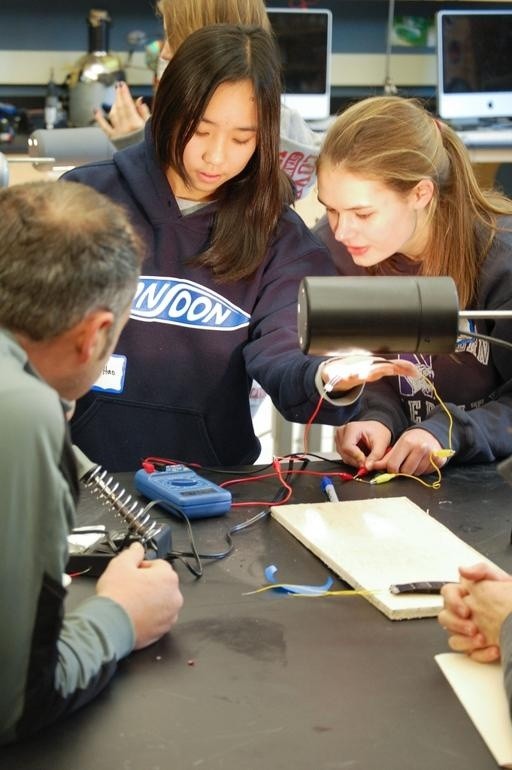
465, 147, 512, 164
0, 459, 512, 770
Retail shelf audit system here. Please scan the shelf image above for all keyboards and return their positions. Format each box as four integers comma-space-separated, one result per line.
455, 129, 512, 147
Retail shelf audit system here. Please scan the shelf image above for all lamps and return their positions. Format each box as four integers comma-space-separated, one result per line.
1, 127, 118, 170
297, 275, 512, 357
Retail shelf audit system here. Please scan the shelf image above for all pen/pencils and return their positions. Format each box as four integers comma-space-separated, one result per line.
320, 474, 341, 501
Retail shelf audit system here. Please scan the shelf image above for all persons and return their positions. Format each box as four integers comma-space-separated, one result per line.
90, 1, 274, 152
57, 19, 420, 484
0, 172, 185, 758
302, 88, 512, 478
434, 562, 511, 722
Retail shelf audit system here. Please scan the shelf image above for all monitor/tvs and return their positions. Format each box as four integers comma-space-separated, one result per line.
434, 9, 512, 129
266, 8, 333, 121
